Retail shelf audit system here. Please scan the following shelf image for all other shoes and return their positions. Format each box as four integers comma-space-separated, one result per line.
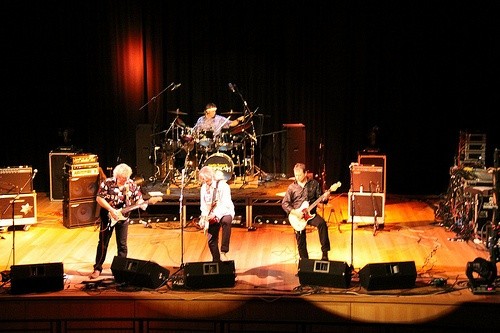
322, 252, 328, 260
220, 252, 228, 261
90, 270, 100, 279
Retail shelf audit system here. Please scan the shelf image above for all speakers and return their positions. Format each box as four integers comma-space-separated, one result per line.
357, 261, 417, 291
281, 124, 307, 178
137, 124, 169, 179
0, 168, 34, 194
10, 262, 64, 294
111, 256, 170, 289
298, 258, 351, 288
350, 165, 383, 192
49, 153, 102, 229
184, 260, 235, 290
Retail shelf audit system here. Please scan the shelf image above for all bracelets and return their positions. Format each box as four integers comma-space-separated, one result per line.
237, 118, 239, 121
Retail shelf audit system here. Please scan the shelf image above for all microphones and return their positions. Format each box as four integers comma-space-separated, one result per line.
229, 83, 235, 93
32, 169, 38, 179
171, 84, 181, 91
182, 169, 186, 175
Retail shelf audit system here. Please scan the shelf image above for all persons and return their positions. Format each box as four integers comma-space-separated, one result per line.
282, 163, 330, 277
199, 166, 235, 262
90, 163, 148, 279
193, 103, 245, 141
486, 167, 500, 173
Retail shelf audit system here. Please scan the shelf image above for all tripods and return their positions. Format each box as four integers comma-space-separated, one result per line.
241, 129, 269, 181
161, 116, 180, 187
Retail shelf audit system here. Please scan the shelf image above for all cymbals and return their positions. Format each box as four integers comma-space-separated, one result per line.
230, 122, 252, 134
222, 112, 243, 114
168, 111, 188, 115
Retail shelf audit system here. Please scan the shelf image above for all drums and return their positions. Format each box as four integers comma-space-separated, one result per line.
180, 128, 194, 141
198, 129, 213, 145
215, 132, 233, 151
202, 152, 234, 180
232, 136, 243, 147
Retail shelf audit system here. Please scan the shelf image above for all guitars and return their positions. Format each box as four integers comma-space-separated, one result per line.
203, 200, 219, 235
288, 180, 342, 232
99, 196, 163, 228
